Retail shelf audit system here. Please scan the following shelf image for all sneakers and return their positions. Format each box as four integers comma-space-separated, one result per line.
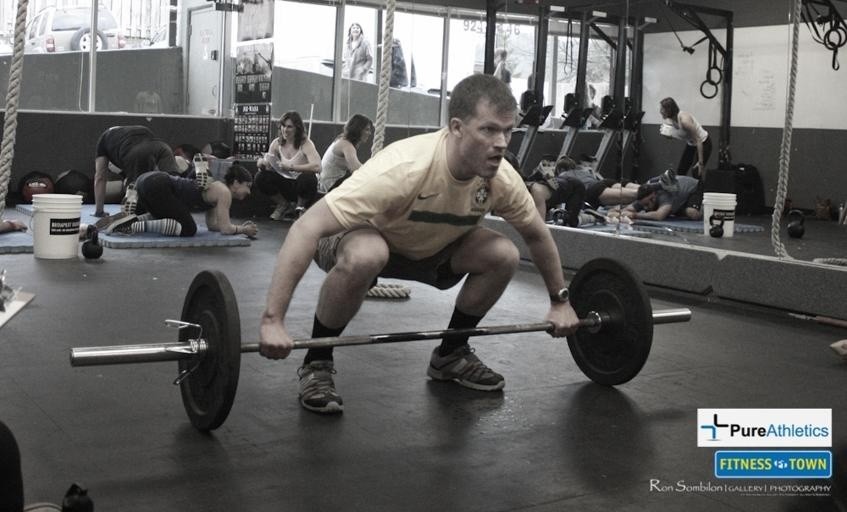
124, 182, 138, 215
585, 209, 607, 226
194, 153, 209, 190
95, 211, 128, 232
270, 201, 292, 221
107, 214, 138, 236
296, 358, 344, 414
428, 343, 505, 391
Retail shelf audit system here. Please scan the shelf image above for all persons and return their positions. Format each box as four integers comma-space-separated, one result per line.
256, 72, 584, 415
22, 17, 715, 242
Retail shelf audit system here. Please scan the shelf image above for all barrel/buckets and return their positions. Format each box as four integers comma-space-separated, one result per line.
701, 191, 739, 239
27, 192, 84, 261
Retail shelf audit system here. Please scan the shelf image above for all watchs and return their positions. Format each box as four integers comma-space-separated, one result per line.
549, 287, 571, 304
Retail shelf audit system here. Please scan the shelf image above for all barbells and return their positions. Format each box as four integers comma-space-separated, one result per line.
71, 258, 692, 433
